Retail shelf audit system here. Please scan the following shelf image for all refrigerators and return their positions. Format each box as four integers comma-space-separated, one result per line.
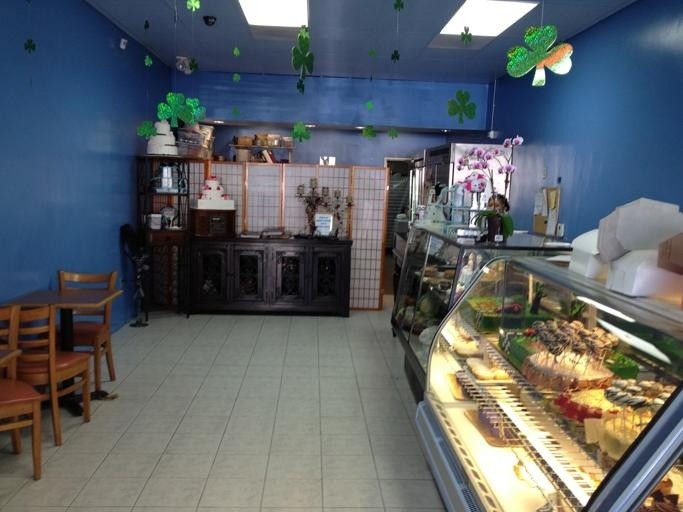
407, 153, 424, 218
423, 143, 512, 229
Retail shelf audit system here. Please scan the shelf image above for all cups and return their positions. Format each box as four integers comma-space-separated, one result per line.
235, 149, 250, 162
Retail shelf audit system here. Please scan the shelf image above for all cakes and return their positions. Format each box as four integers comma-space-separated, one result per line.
195, 175, 230, 200
146, 118, 177, 155
447, 316, 683, 512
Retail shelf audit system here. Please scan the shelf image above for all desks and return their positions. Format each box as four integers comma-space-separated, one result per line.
131, 238, 192, 326
2, 284, 124, 418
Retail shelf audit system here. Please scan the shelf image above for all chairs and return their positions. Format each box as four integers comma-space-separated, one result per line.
0, 303, 47, 481
46, 267, 118, 395
14, 301, 93, 449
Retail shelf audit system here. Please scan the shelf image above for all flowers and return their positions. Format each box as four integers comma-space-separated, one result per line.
459, 145, 498, 195
490, 134, 525, 197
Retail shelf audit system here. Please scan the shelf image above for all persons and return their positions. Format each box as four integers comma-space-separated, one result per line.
488, 194, 513, 237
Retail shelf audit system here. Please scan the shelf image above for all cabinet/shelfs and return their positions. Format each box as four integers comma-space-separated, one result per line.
178, 238, 229, 317
132, 154, 193, 235
229, 236, 307, 317
409, 246, 681, 512
307, 237, 353, 319
391, 222, 575, 407
392, 216, 415, 307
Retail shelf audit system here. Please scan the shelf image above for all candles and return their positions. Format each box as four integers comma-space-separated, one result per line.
295, 176, 355, 215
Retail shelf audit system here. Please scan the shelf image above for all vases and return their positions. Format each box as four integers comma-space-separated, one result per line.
485, 217, 508, 243
